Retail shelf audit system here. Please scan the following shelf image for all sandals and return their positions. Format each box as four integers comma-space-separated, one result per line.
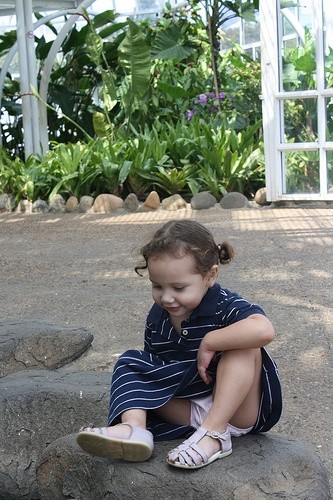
76, 423, 153, 463
164, 426, 232, 470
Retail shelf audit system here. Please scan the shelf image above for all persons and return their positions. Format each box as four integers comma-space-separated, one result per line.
76, 218, 285, 470
